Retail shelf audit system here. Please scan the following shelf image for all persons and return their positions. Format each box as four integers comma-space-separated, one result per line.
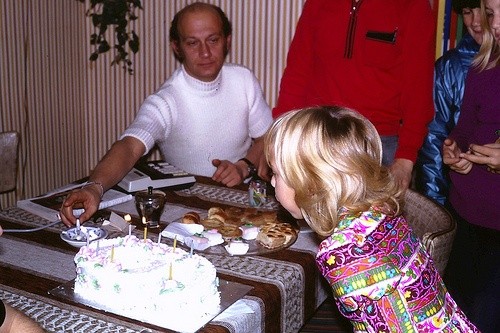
272, 0, 438, 198
265, 105, 480, 333
0, 299, 45, 333
60, 3, 273, 228
425, 0, 500, 333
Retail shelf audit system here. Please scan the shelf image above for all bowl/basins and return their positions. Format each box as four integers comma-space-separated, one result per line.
59, 226, 109, 247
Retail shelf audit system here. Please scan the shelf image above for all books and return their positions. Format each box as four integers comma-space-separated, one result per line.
17, 183, 132, 221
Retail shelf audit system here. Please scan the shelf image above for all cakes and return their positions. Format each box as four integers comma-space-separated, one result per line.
73, 234, 221, 330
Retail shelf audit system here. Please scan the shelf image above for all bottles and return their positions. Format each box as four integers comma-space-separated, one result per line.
247, 175, 270, 209
135, 186, 166, 227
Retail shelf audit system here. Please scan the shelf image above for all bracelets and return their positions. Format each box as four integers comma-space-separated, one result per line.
82, 182, 104, 198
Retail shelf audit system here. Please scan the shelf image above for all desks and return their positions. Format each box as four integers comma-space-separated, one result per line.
0, 173, 328, 333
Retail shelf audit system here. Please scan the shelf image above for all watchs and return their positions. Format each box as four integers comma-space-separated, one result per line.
238, 158, 256, 178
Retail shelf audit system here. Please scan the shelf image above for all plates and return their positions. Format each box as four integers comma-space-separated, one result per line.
167, 211, 298, 256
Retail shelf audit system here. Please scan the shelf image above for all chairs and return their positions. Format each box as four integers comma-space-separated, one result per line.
299, 187, 459, 333
0, 130, 19, 209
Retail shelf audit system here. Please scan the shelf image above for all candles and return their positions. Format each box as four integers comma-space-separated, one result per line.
129, 224, 132, 234
111, 244, 114, 263
142, 216, 147, 240
173, 235, 177, 253
169, 262, 173, 281
157, 225, 161, 243
86, 235, 90, 248
190, 239, 193, 258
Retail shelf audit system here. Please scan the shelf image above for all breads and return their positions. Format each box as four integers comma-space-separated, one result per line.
182, 207, 296, 248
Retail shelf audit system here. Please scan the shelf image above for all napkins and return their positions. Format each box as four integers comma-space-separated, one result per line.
161, 222, 204, 242
